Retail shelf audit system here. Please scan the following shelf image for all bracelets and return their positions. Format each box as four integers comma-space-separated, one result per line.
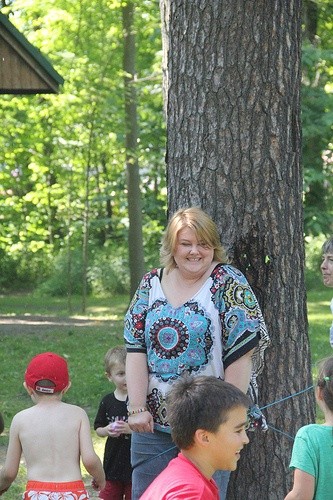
126, 406, 148, 417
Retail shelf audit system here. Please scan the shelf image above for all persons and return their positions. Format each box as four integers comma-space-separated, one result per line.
281, 356, 333, 500
0, 350, 107, 500
93, 344, 138, 500
136, 371, 251, 500
122, 206, 272, 500
319, 233, 333, 349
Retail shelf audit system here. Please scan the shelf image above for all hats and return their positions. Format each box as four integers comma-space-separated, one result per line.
24, 352, 69, 393
